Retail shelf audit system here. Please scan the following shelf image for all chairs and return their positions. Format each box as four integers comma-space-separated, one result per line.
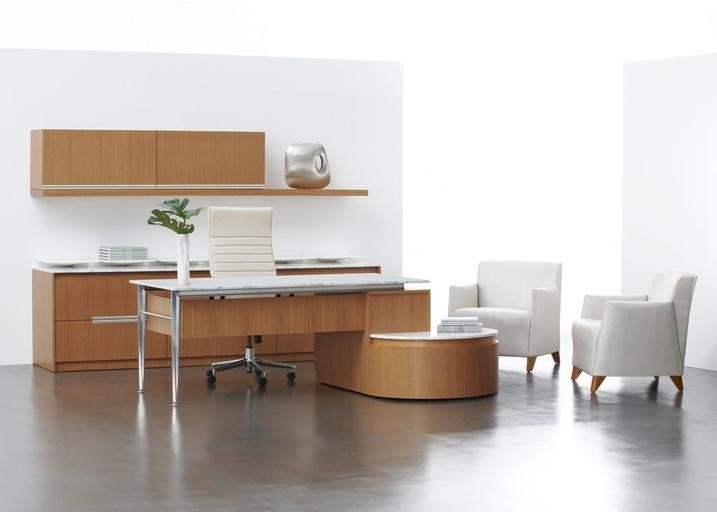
198, 205, 298, 388
569, 269, 699, 395
444, 258, 564, 372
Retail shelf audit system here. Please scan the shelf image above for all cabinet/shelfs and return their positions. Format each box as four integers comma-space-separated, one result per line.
26, 126, 368, 200
25, 258, 382, 376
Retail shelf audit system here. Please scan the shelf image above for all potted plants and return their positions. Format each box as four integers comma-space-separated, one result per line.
143, 197, 206, 286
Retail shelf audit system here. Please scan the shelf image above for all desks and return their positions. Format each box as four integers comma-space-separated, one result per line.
124, 270, 501, 407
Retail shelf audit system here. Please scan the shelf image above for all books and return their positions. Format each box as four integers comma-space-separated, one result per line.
98, 245, 149, 262
436, 316, 482, 334
